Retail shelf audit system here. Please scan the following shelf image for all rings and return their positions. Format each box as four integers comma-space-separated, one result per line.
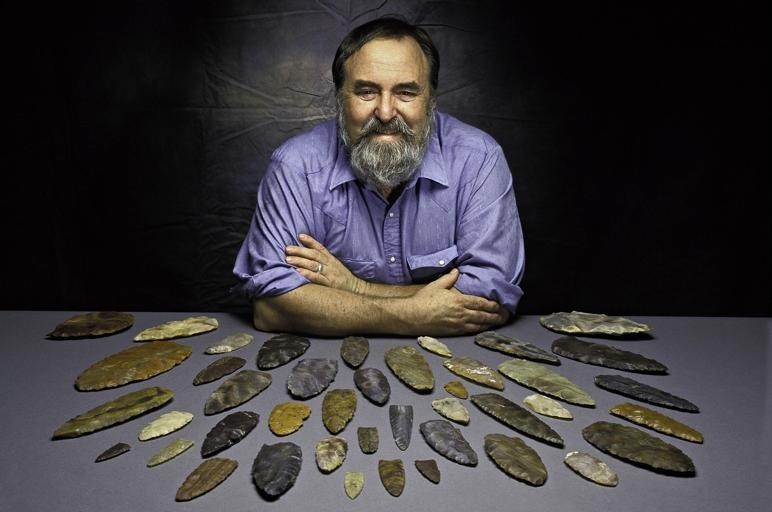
318, 262, 325, 274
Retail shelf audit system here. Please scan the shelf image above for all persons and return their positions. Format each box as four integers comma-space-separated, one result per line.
231, 12, 529, 339
